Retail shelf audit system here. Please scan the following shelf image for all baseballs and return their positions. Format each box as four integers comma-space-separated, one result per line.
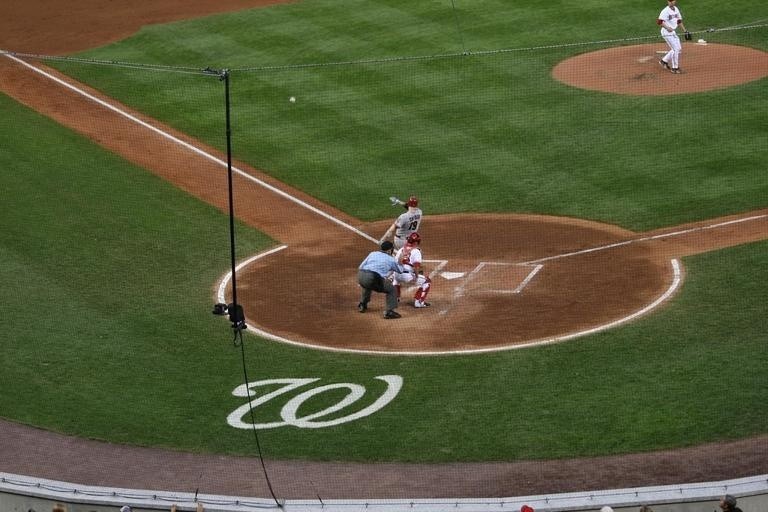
289, 97, 297, 103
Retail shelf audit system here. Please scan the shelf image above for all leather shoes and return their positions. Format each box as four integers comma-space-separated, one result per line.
383, 310, 401, 319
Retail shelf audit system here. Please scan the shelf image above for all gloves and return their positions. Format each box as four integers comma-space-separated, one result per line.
389, 196, 400, 206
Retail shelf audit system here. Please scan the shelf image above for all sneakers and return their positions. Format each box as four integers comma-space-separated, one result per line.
659, 60, 680, 74
359, 303, 367, 313
397, 297, 401, 304
414, 299, 432, 308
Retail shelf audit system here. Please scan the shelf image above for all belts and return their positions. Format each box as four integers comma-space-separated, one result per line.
403, 271, 409, 273
395, 234, 408, 240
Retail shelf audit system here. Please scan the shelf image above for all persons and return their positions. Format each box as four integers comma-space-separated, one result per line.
720, 494, 743, 512
356, 241, 404, 320
119, 505, 132, 512
170, 502, 205, 511
521, 505, 533, 512
391, 233, 432, 307
378, 194, 422, 249
656, 0, 690, 74
639, 505, 653, 512
51, 504, 67, 512
599, 506, 614, 512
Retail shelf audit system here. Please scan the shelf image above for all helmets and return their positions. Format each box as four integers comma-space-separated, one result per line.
408, 197, 418, 207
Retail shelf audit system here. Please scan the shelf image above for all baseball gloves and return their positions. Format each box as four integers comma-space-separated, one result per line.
685, 30, 691, 39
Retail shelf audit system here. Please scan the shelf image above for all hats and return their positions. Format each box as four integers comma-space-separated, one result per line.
381, 241, 393, 251
